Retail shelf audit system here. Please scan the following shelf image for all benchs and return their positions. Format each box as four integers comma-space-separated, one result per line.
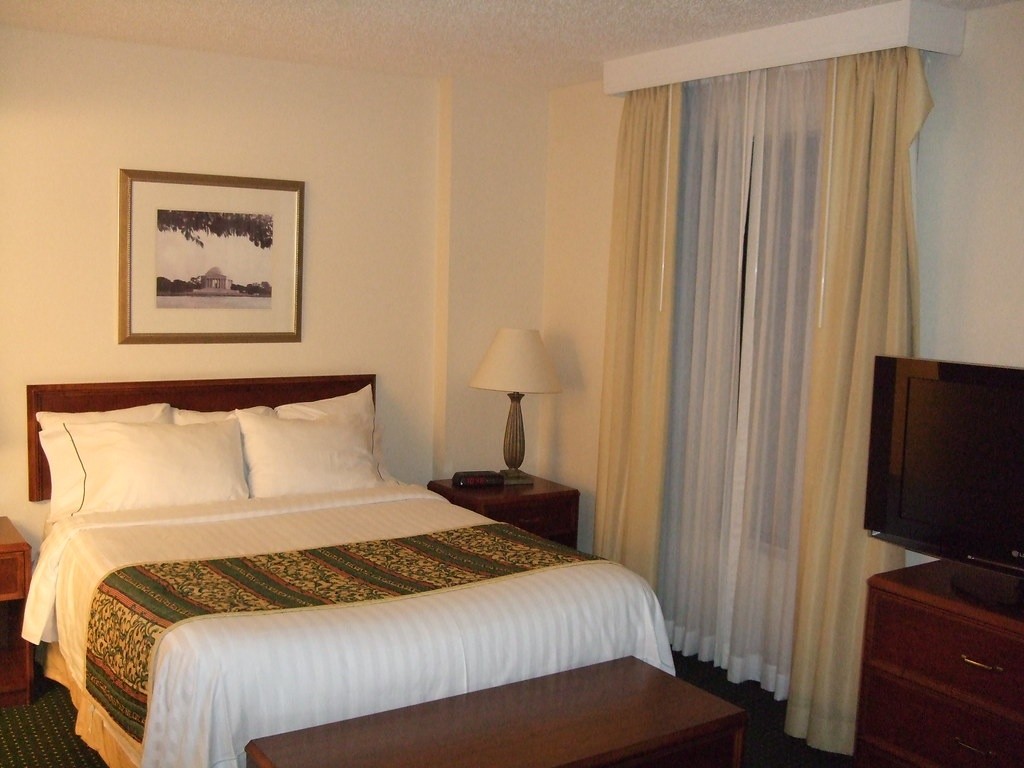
244, 655, 749, 768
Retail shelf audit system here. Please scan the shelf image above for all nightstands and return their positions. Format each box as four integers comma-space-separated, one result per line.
0, 516, 32, 707
427, 475, 580, 551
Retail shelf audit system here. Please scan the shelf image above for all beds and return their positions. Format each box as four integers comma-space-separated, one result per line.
20, 374, 676, 768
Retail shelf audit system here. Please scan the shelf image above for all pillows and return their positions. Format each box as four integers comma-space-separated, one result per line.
37, 418, 249, 523
173, 405, 277, 427
276, 383, 376, 449
36, 404, 173, 431
236, 408, 399, 497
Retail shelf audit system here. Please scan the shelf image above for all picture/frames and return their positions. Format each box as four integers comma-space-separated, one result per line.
118, 168, 305, 343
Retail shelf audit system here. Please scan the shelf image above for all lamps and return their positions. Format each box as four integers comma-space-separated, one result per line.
468, 328, 562, 485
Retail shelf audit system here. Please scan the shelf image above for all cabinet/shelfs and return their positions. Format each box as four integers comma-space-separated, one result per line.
853, 559, 1024, 768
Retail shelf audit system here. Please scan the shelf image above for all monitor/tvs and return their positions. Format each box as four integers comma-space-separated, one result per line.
863, 355, 1024, 612
